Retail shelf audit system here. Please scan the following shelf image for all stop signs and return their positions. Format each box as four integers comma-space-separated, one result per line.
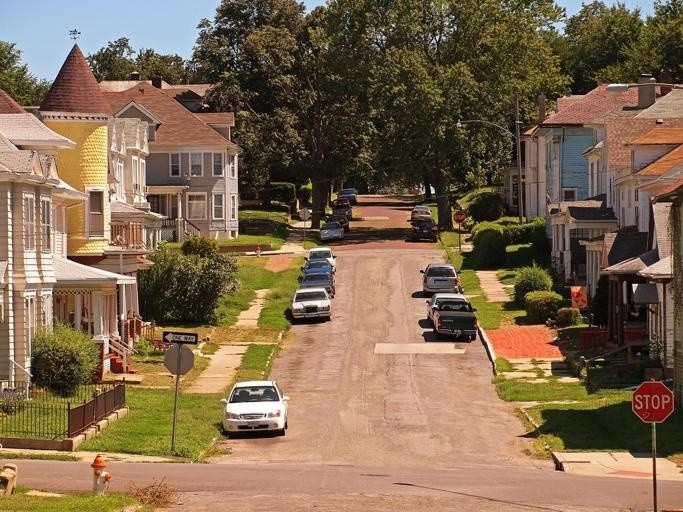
455, 212, 465, 223
632, 382, 675, 424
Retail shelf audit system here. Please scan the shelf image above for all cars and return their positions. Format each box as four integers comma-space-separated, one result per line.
291, 286, 332, 319
410, 205, 432, 221
320, 188, 359, 241
298, 247, 338, 298
220, 380, 290, 437
425, 293, 468, 320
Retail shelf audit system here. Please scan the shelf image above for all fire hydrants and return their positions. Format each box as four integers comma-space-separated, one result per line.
91, 454, 112, 497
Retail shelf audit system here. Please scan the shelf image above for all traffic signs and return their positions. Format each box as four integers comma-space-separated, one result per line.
162, 332, 198, 344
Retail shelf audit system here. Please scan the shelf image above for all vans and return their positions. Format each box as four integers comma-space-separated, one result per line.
431, 299, 478, 341
410, 217, 439, 242
419, 263, 462, 296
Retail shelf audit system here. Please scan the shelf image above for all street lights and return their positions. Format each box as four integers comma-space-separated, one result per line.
604, 83, 682, 94
456, 93, 522, 225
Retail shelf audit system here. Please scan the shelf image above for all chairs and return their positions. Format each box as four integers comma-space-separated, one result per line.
460, 307, 467, 311
262, 389, 275, 400
238, 390, 249, 401
444, 306, 450, 311
